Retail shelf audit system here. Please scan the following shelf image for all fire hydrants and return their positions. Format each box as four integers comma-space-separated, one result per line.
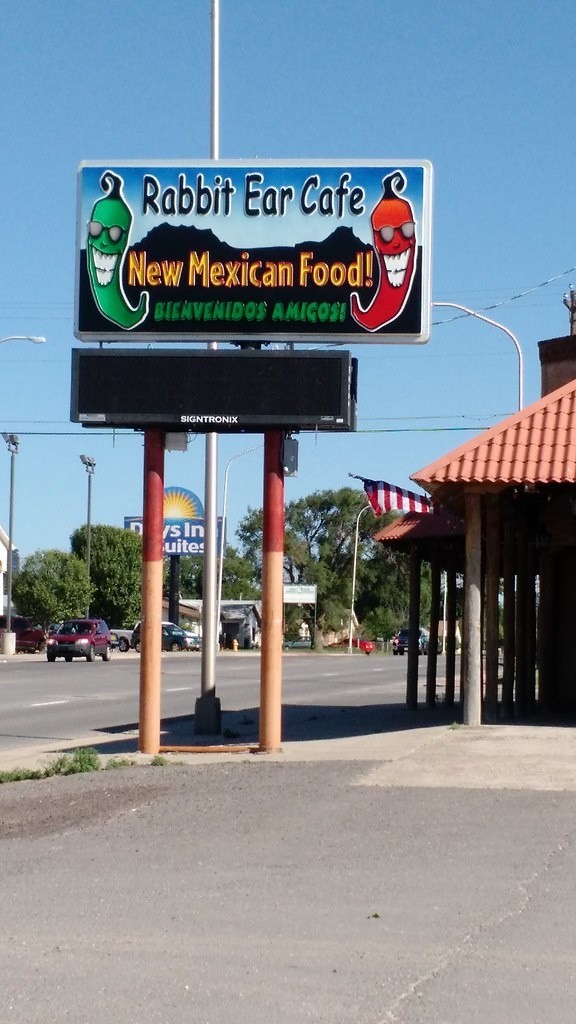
232, 639, 239, 652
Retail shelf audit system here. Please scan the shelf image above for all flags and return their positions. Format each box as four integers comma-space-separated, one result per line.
363, 479, 431, 518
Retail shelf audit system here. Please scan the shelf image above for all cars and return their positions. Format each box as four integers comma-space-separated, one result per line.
283, 636, 311, 651
323, 638, 375, 655
131, 624, 199, 653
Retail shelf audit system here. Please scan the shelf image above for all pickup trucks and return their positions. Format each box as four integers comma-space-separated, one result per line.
110, 621, 197, 652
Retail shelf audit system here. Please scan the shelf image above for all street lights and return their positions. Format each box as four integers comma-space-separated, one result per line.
1, 431, 20, 657
79, 453, 96, 619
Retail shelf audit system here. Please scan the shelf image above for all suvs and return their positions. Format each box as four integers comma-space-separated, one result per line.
392, 627, 429, 655
47, 619, 112, 662
